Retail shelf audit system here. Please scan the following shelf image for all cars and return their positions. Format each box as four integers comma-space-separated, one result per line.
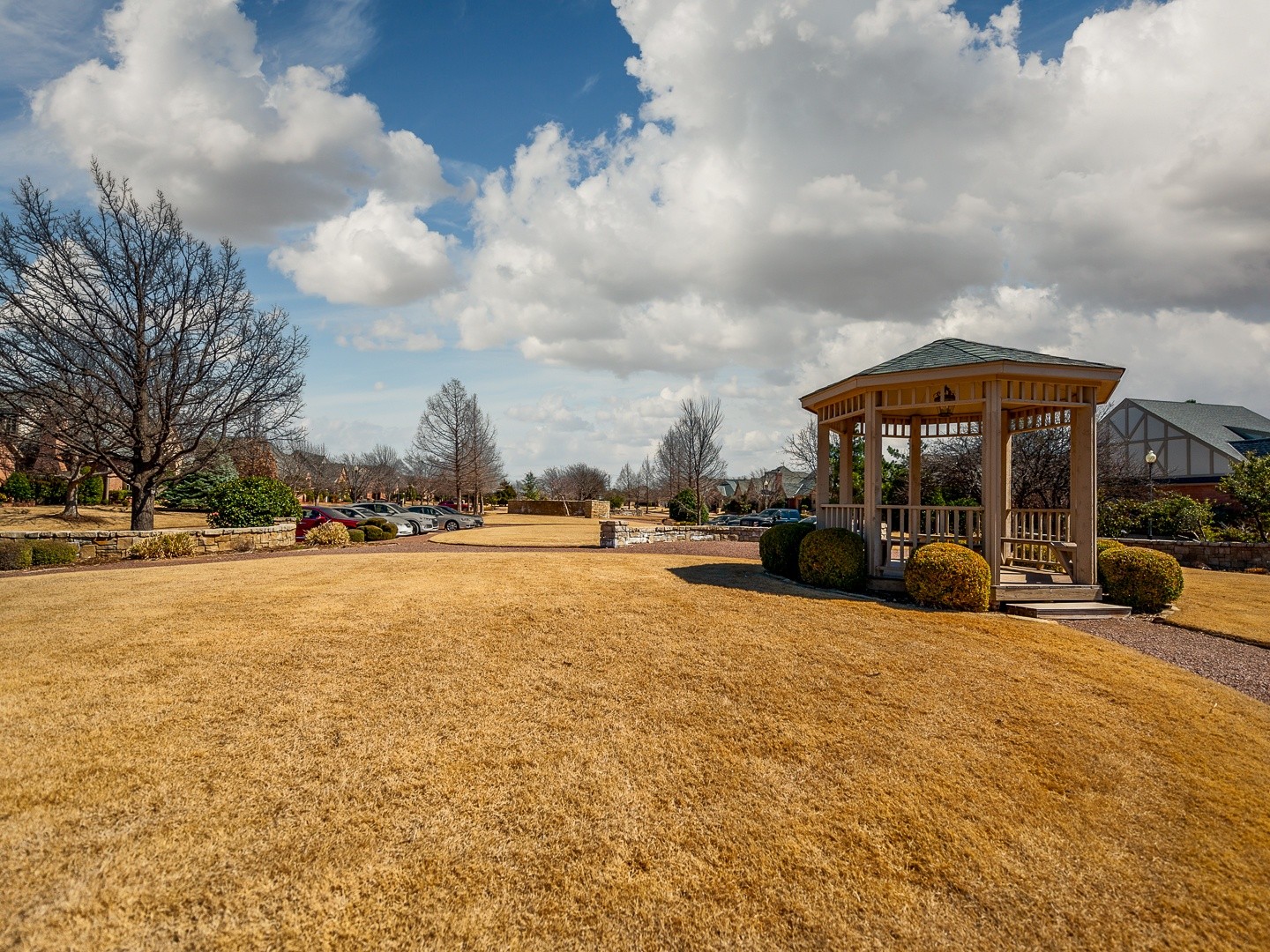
437, 500, 472, 512
296, 506, 413, 542
799, 516, 817, 525
708, 514, 758, 526
404, 506, 483, 532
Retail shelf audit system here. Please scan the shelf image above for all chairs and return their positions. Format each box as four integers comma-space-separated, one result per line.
302, 510, 307, 517
309, 510, 315, 518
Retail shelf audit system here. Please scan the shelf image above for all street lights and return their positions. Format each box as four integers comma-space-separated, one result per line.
1145, 450, 1157, 540
764, 480, 769, 509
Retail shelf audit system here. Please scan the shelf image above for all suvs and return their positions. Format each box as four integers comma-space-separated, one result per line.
340, 501, 439, 536
740, 509, 799, 527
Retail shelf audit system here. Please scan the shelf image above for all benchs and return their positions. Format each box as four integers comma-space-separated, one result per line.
879, 533, 1076, 583
